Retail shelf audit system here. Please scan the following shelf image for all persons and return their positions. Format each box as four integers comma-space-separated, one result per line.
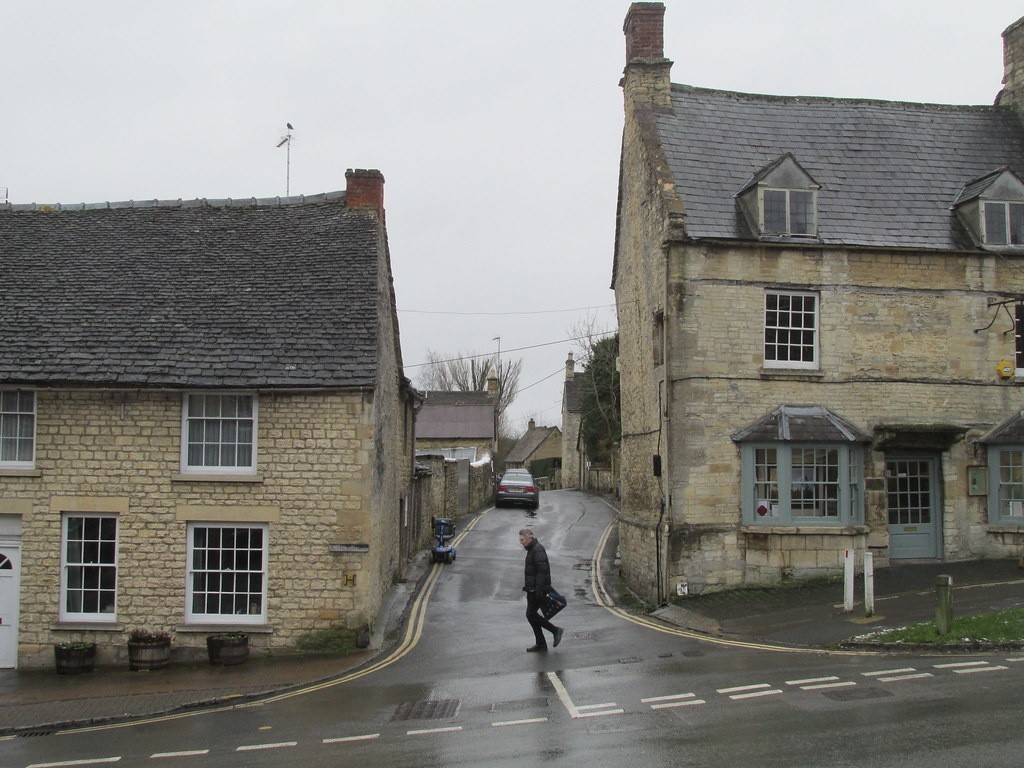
519, 529, 563, 652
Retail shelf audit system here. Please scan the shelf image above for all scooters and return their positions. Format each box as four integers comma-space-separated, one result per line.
430, 516, 456, 564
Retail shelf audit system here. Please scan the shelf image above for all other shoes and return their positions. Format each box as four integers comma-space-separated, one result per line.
553, 627, 563, 647
527, 644, 547, 652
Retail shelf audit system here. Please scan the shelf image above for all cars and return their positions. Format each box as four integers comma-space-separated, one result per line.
495, 468, 541, 509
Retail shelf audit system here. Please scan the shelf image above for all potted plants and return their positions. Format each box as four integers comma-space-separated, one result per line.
54, 643, 96, 670
207, 631, 250, 666
126, 630, 172, 669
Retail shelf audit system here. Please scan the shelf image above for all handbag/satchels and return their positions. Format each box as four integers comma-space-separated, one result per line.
540, 587, 567, 620
